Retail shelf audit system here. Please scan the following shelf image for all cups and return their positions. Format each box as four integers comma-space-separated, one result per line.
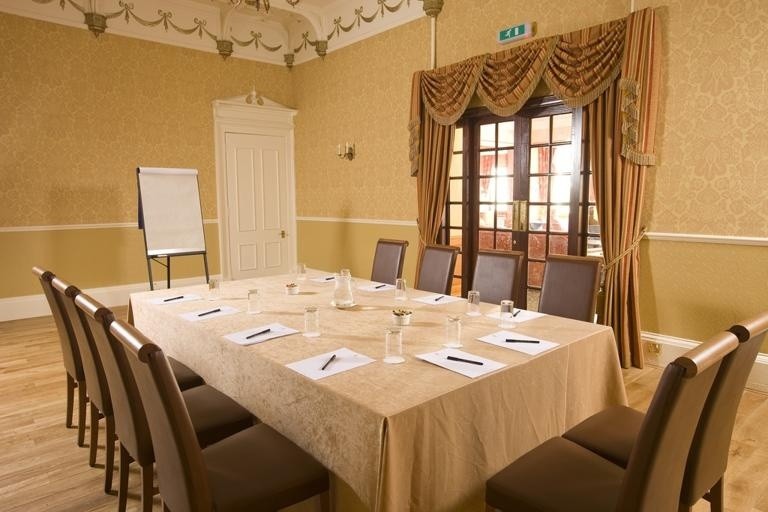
207, 274, 220, 301
498, 299, 516, 328
246, 288, 261, 315
304, 305, 322, 338
385, 329, 404, 359
447, 318, 461, 346
341, 267, 351, 279
296, 262, 310, 282
467, 290, 480, 316
394, 278, 406, 301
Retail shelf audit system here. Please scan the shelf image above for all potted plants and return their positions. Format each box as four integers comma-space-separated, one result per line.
284, 283, 299, 296
391, 309, 412, 327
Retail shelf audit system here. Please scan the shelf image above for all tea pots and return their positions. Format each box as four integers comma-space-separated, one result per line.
333, 275, 357, 309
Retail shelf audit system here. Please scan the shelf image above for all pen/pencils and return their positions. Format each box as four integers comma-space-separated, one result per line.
322, 355, 336, 370
163, 296, 184, 302
447, 355, 483, 365
326, 277, 335, 280
506, 339, 539, 343
435, 296, 444, 301
376, 285, 386, 288
513, 310, 520, 317
199, 309, 221, 316
246, 328, 270, 338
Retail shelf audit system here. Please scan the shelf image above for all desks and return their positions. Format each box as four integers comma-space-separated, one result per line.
127, 266, 631, 511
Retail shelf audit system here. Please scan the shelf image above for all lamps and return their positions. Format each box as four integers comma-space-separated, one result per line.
334, 136, 358, 161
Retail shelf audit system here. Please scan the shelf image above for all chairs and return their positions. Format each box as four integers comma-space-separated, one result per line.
410, 245, 458, 297
107, 319, 331, 511
50, 276, 206, 512
29, 264, 91, 448
571, 310, 766, 512
534, 248, 602, 324
72, 294, 255, 512
468, 246, 523, 311
368, 237, 411, 287
481, 330, 740, 512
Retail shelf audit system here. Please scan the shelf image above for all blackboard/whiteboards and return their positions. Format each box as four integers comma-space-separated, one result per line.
136, 167, 206, 258
